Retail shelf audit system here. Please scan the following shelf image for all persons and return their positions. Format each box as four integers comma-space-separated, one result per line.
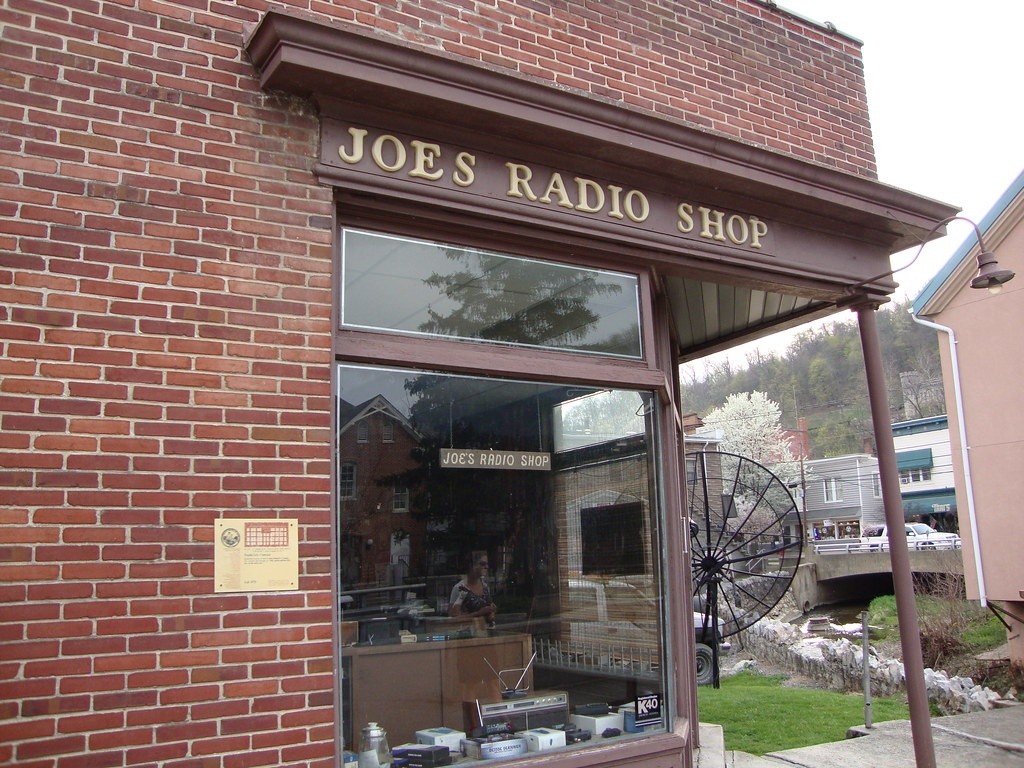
448, 549, 514, 738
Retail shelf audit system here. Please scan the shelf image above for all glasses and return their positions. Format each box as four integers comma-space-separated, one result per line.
474, 561, 488, 566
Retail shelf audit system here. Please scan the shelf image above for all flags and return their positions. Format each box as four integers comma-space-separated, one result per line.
930, 515, 938, 529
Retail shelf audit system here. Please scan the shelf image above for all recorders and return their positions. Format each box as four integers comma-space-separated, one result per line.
462, 651, 569, 737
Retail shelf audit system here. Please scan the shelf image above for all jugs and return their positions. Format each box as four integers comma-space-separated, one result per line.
358, 722, 390, 768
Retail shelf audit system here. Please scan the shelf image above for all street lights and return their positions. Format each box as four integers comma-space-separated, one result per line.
788, 481, 807, 548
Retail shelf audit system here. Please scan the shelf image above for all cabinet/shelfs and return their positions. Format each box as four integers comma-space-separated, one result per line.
339, 629, 535, 756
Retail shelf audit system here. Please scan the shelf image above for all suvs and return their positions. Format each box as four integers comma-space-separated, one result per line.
566, 578, 731, 686
858, 522, 962, 552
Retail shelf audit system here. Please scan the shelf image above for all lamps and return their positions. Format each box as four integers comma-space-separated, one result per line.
842, 215, 1015, 297
